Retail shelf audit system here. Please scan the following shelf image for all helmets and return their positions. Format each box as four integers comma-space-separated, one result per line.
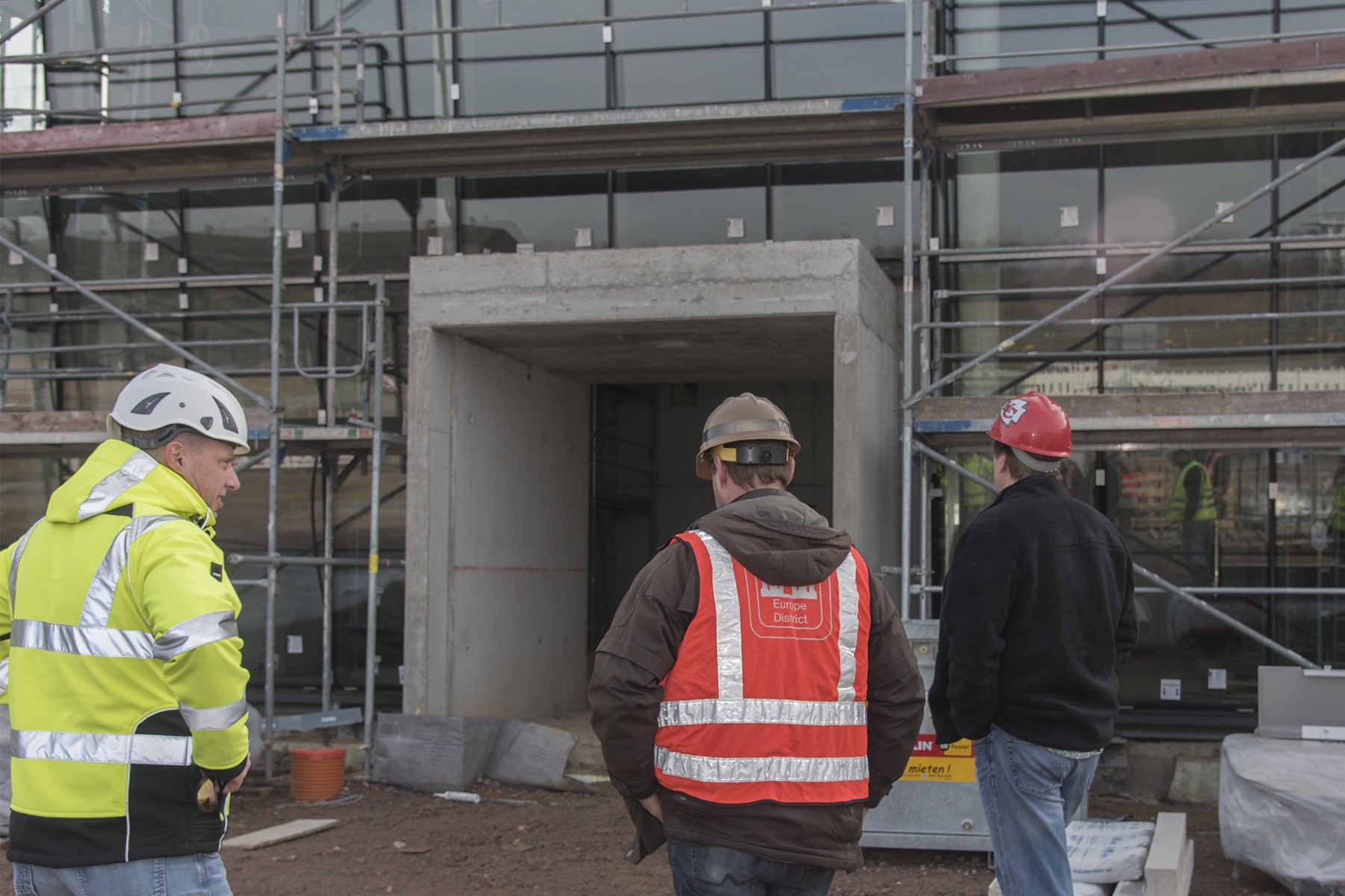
695, 394, 800, 479
983, 392, 1071, 474
105, 362, 253, 458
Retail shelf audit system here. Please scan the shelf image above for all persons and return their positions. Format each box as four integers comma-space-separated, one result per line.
0, 362, 253, 896
928, 391, 1136, 896
589, 392, 925, 896
937, 454, 995, 530
1060, 448, 1235, 599
